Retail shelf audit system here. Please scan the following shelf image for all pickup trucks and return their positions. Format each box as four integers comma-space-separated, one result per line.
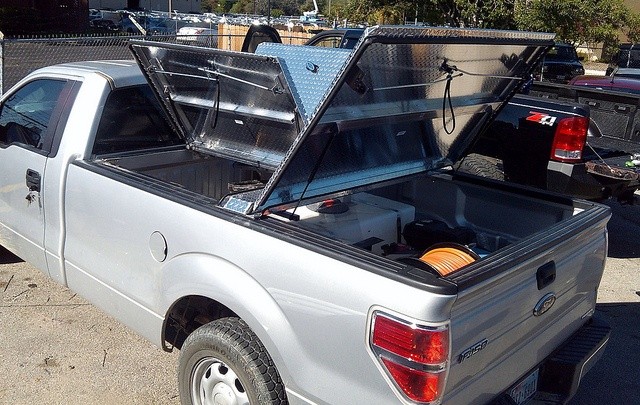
605, 43, 640, 79
0, 23, 612, 405
240, 22, 640, 206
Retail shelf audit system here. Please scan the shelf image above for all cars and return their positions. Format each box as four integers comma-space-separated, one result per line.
176, 21, 219, 48
566, 74, 640, 94
219, 9, 330, 29
116, 16, 157, 33
116, 9, 219, 20
145, 18, 188, 36
89, 7, 115, 30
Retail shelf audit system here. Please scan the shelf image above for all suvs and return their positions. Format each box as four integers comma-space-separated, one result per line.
531, 41, 585, 85
